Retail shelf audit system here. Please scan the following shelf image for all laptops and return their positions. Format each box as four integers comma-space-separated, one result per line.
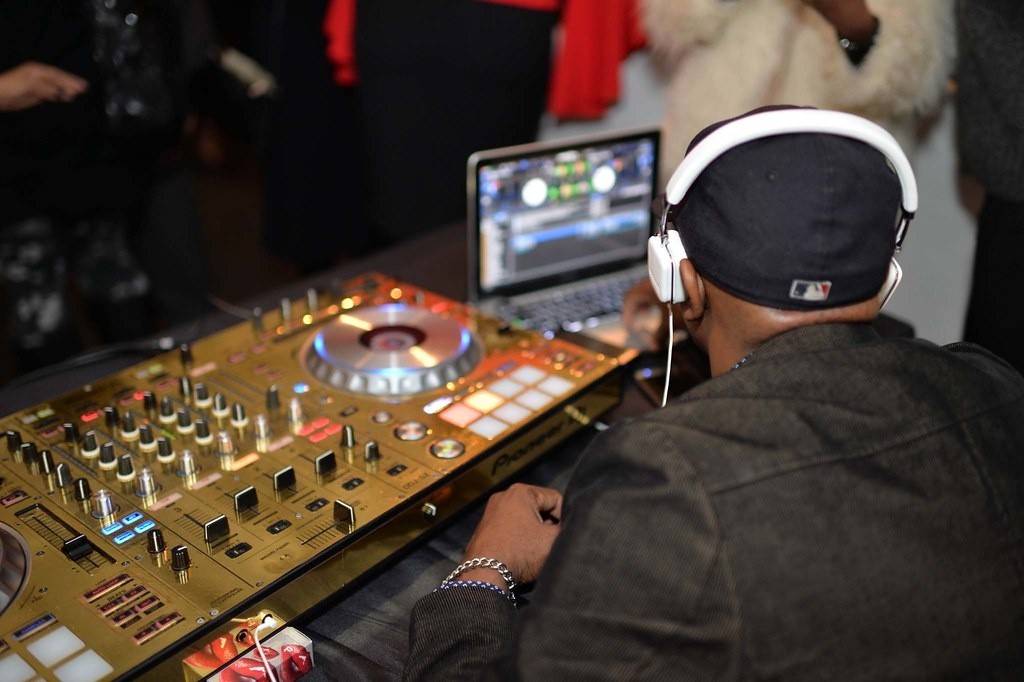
463, 124, 659, 337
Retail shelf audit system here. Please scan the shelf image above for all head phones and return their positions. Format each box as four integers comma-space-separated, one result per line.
646, 108, 919, 314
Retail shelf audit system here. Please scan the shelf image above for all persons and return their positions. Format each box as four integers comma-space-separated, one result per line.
639, 0, 952, 196
399, 102, 1024, 681
0, 0, 201, 380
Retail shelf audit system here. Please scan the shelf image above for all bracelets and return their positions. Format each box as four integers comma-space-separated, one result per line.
441, 558, 517, 608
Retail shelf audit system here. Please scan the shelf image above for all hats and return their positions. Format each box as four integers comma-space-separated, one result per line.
678, 104, 902, 313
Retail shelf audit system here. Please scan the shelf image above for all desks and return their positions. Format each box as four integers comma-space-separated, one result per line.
1, 217, 712, 681
